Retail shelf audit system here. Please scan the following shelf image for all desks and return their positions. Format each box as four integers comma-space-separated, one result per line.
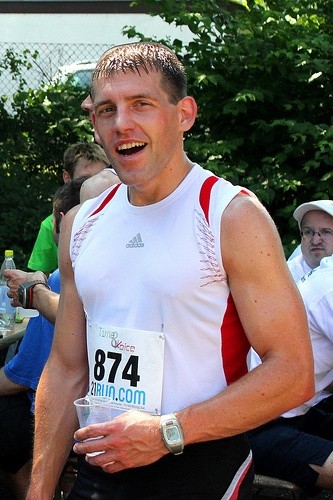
0, 316, 30, 368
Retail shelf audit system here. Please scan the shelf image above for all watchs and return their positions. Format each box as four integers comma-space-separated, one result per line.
18, 280, 51, 311
160, 412, 184, 456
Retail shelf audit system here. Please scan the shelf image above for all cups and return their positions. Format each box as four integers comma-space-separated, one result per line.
73, 396, 112, 458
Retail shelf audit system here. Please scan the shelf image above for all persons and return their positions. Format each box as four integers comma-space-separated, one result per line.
0, 41, 332, 500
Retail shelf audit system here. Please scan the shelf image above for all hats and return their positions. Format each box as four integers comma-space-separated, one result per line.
293, 200, 332, 230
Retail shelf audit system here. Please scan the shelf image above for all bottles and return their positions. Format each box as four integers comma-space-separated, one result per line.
0, 250, 16, 331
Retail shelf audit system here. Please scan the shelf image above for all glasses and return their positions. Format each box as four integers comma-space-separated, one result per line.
299, 226, 332, 239
